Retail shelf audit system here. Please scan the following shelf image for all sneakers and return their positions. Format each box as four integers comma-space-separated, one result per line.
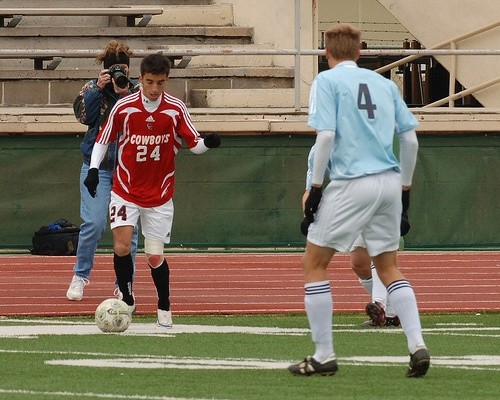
113, 288, 135, 301
66, 274, 89, 301
365, 303, 385, 326
288, 356, 339, 377
128, 296, 136, 313
405, 349, 430, 378
156, 308, 173, 328
362, 316, 400, 327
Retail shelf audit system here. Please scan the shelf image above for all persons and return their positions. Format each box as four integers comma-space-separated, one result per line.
288, 25, 431, 378
82, 55, 221, 328
65, 41, 140, 301
302, 144, 410, 326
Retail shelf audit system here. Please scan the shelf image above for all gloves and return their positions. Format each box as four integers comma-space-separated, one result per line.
401, 189, 410, 211
400, 211, 410, 236
300, 216, 309, 237
302, 186, 322, 222
204, 132, 221, 148
83, 168, 100, 198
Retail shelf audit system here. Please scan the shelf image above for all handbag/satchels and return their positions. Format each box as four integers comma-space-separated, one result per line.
29, 218, 81, 255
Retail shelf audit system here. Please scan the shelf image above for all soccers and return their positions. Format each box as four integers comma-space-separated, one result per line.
95, 298, 131, 333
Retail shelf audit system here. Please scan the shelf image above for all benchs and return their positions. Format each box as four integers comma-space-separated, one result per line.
0, 7, 199, 69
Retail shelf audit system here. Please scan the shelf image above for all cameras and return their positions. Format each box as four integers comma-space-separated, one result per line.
108, 65, 128, 88
